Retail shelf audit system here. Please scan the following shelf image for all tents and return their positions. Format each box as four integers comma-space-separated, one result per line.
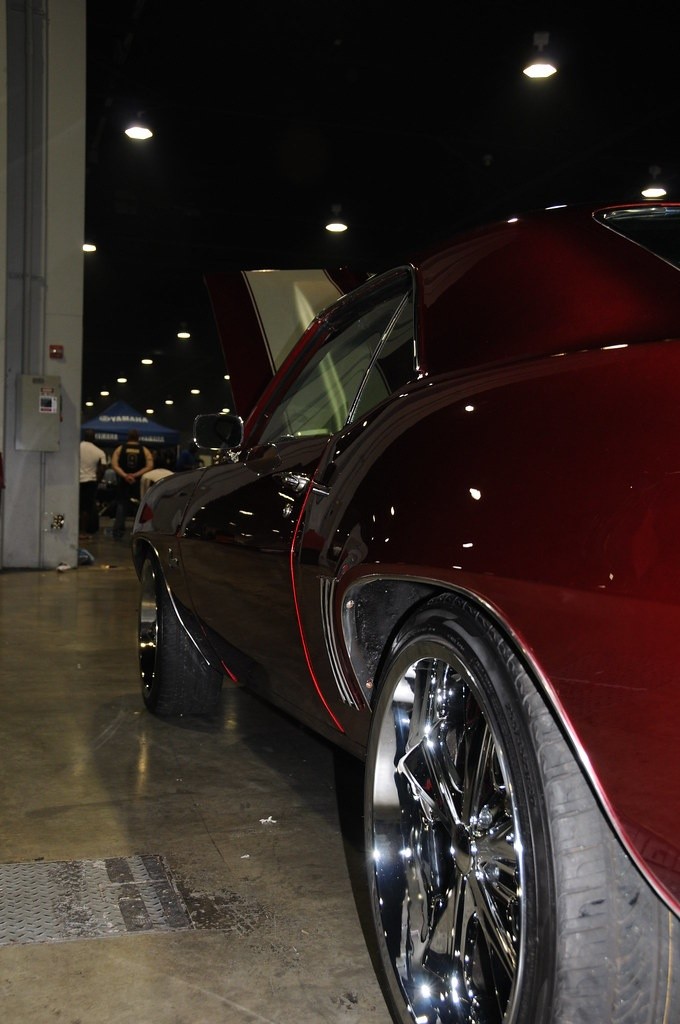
81, 402, 178, 470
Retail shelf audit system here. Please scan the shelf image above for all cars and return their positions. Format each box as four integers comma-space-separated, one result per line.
124, 194, 680, 1024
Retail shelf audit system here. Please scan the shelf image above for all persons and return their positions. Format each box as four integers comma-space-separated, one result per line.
177, 442, 199, 473
111, 429, 152, 541
78, 431, 108, 541
